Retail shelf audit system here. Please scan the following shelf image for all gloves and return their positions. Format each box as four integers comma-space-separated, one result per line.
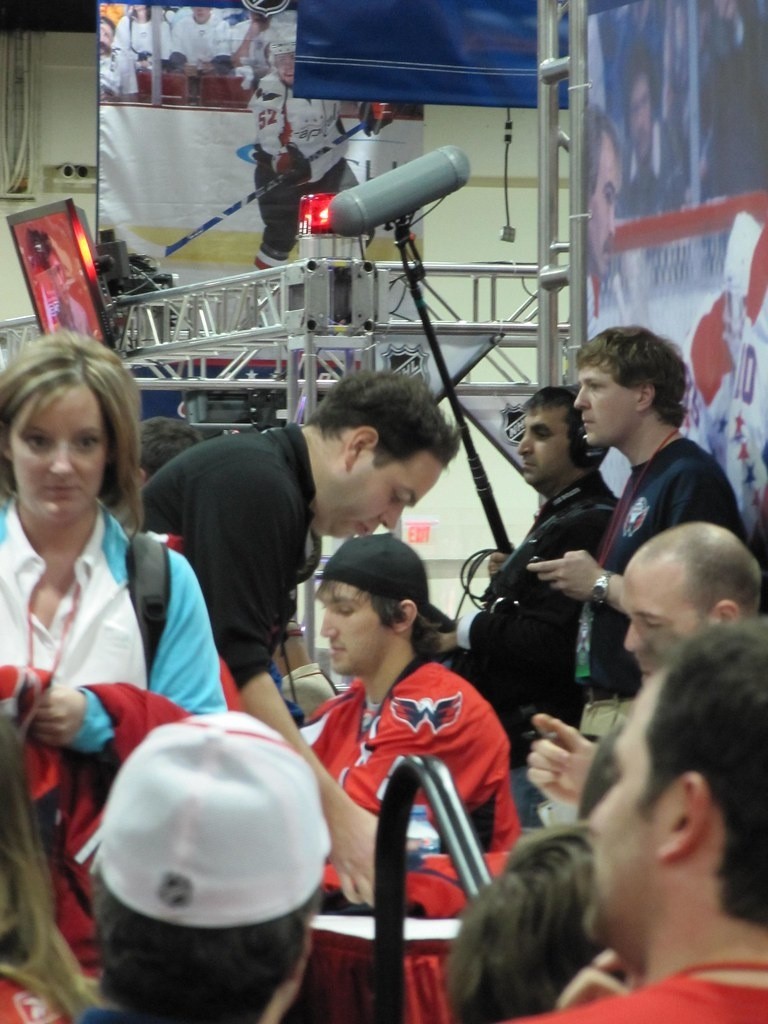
270, 144, 312, 188
356, 101, 397, 137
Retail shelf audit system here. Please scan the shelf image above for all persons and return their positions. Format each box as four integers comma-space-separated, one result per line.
100, 2, 768, 545
1, 325, 768, 1024
308, 535, 519, 917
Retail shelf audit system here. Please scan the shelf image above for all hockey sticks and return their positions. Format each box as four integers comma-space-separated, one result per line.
110, 122, 368, 260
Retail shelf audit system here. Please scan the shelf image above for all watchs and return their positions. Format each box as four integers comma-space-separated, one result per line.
592, 568, 615, 601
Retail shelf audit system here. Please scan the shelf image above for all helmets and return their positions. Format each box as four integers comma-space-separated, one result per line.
268, 21, 297, 55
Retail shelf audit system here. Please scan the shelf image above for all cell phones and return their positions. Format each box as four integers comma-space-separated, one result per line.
529, 556, 545, 563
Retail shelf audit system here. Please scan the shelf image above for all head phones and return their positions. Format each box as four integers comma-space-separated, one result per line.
562, 385, 606, 467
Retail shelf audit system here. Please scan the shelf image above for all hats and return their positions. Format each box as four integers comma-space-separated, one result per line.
73, 711, 332, 928
322, 532, 457, 633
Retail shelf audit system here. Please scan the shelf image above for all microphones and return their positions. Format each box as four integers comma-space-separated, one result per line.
328, 145, 471, 237
